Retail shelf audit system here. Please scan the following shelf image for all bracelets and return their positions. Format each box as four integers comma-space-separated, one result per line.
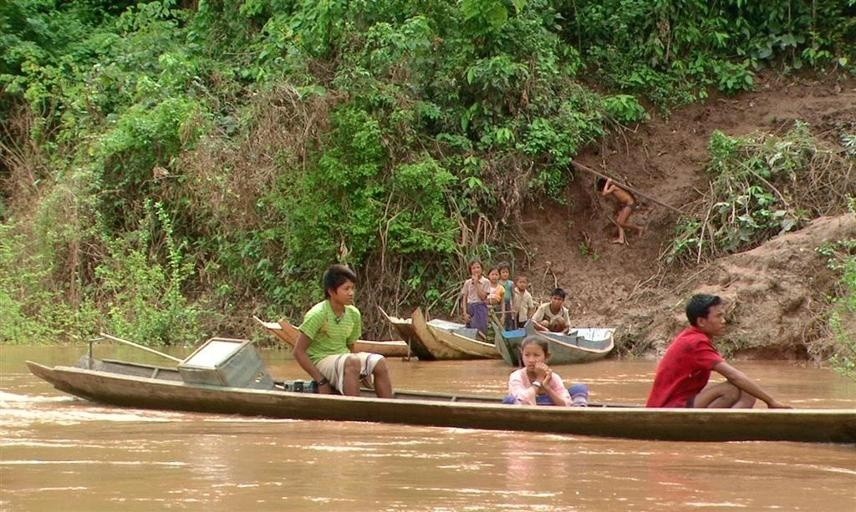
532, 380, 543, 389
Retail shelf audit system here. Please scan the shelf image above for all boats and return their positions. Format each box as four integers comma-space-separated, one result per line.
524, 319, 617, 361
490, 319, 527, 369
376, 305, 501, 362
252, 315, 416, 358
25, 350, 856, 442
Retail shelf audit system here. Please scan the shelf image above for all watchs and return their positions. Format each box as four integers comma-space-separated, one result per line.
316, 376, 327, 387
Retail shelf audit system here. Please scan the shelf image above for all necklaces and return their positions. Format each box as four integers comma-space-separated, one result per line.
332, 305, 345, 324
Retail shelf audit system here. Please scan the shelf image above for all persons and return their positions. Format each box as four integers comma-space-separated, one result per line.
531, 288, 571, 334
503, 334, 588, 407
462, 260, 535, 339
645, 294, 790, 408
598, 178, 645, 245
293, 263, 393, 399
